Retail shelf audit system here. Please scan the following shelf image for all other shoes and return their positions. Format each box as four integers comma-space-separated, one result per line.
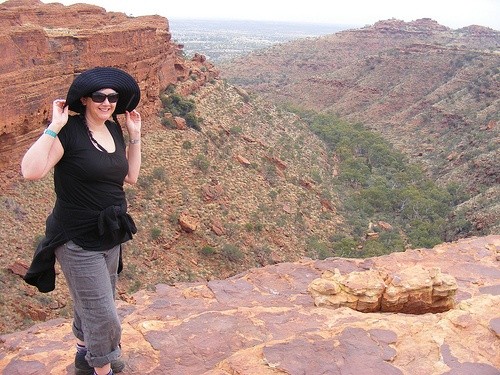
75, 351, 124, 373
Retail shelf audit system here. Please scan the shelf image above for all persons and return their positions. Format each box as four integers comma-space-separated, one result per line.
21, 67, 141, 375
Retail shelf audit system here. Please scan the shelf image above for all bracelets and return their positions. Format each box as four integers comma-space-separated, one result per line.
129, 139, 141, 144
43, 129, 57, 138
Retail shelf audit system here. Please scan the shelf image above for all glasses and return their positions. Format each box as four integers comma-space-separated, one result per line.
88, 92, 119, 103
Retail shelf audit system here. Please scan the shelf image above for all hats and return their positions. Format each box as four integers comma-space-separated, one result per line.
65, 67, 141, 114
